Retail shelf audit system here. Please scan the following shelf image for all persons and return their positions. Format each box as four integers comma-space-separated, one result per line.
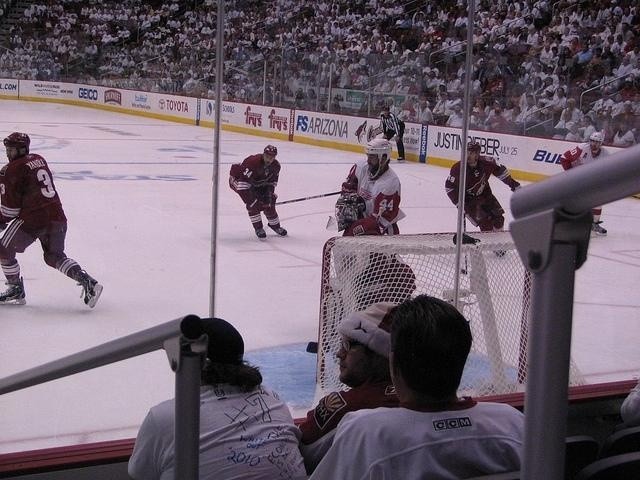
378, 105, 405, 161
127, 318, 307, 480
347, 140, 407, 233
560, 131, 608, 234
445, 142, 521, 257
0, 133, 99, 304
308, 294, 525, 480
336, 190, 414, 310
621, 384, 640, 427
298, 302, 401, 445
229, 145, 288, 238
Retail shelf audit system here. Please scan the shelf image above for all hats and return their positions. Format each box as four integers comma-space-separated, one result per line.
341, 301, 400, 358
201, 318, 245, 364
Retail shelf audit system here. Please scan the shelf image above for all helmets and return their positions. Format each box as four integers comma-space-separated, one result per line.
380, 108, 391, 115
4, 131, 30, 147
590, 132, 605, 143
468, 141, 481, 152
366, 138, 391, 162
264, 145, 277, 156
343, 218, 381, 237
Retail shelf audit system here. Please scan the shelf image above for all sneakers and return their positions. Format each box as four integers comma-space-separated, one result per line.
0, 275, 26, 302
78, 270, 98, 304
590, 221, 607, 233
269, 224, 287, 235
255, 227, 266, 238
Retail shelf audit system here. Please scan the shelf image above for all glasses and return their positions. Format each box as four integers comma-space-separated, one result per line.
338, 340, 360, 352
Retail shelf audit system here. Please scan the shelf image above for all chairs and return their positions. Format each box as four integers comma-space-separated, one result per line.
605, 426, 639, 459
565, 434, 604, 480
577, 451, 640, 480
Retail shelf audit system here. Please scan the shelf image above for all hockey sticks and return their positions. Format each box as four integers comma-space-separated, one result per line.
306, 342, 330, 353
460, 212, 467, 275
